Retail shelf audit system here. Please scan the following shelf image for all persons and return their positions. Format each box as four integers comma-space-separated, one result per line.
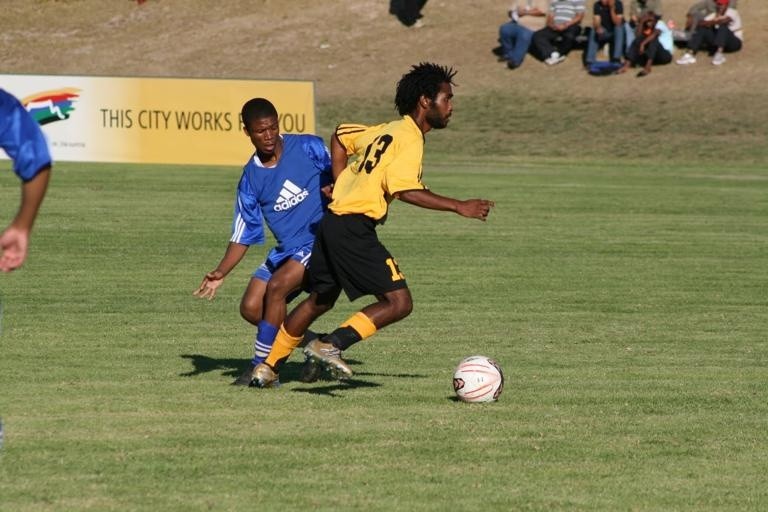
0, 87, 52, 272
250, 63, 495, 389
491, 0, 744, 77
192, 99, 333, 389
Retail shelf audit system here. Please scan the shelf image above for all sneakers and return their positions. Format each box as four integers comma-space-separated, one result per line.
493, 51, 652, 78
231, 333, 354, 389
712, 51, 726, 65
676, 53, 696, 65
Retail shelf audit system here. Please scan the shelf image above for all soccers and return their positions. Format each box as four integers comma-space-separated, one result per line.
454, 357, 504, 403
